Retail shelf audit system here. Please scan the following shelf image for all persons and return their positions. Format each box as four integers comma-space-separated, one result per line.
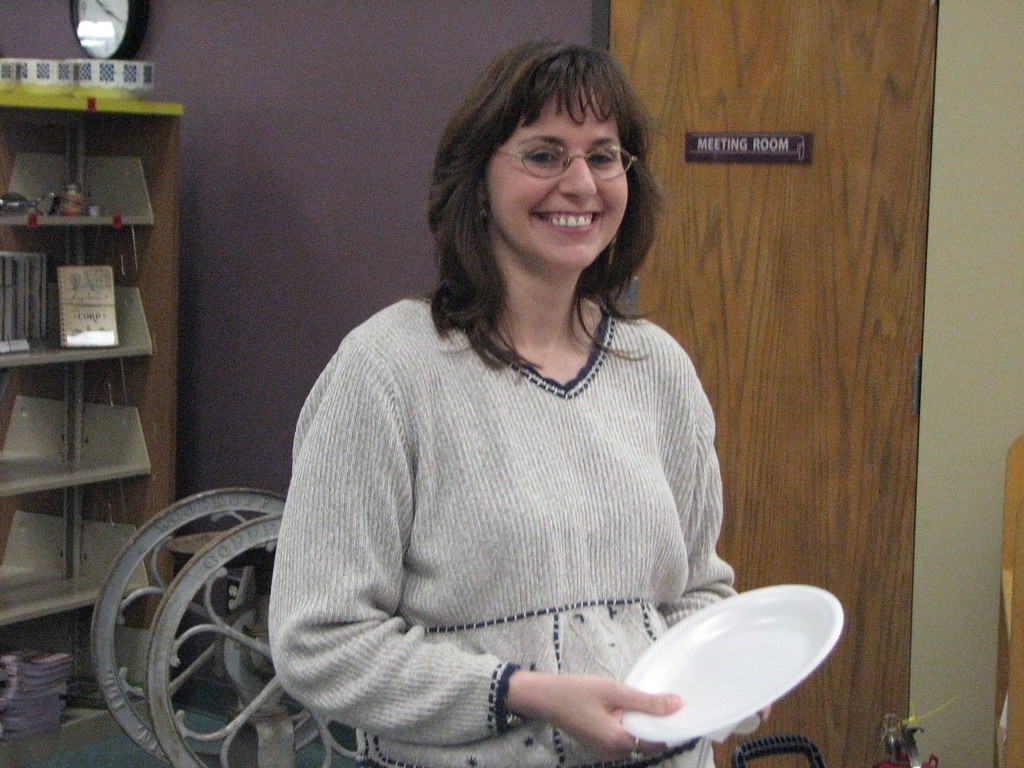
269, 37, 771, 768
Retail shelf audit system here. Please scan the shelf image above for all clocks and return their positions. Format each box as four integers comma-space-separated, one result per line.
68, 0, 150, 61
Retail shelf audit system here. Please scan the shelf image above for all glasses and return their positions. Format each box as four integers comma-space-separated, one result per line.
496, 143, 638, 181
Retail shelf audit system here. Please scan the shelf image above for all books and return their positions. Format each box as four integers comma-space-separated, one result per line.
58, 265, 118, 348
0, 251, 48, 342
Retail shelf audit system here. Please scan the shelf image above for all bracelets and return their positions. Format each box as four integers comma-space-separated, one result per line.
504, 713, 524, 727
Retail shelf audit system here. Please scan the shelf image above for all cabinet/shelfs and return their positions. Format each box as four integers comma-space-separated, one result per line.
0, 94, 187, 768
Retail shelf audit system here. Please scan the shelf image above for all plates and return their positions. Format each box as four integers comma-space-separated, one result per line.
618, 584, 844, 750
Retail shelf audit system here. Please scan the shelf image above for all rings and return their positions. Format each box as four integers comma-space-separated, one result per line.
632, 738, 643, 760
